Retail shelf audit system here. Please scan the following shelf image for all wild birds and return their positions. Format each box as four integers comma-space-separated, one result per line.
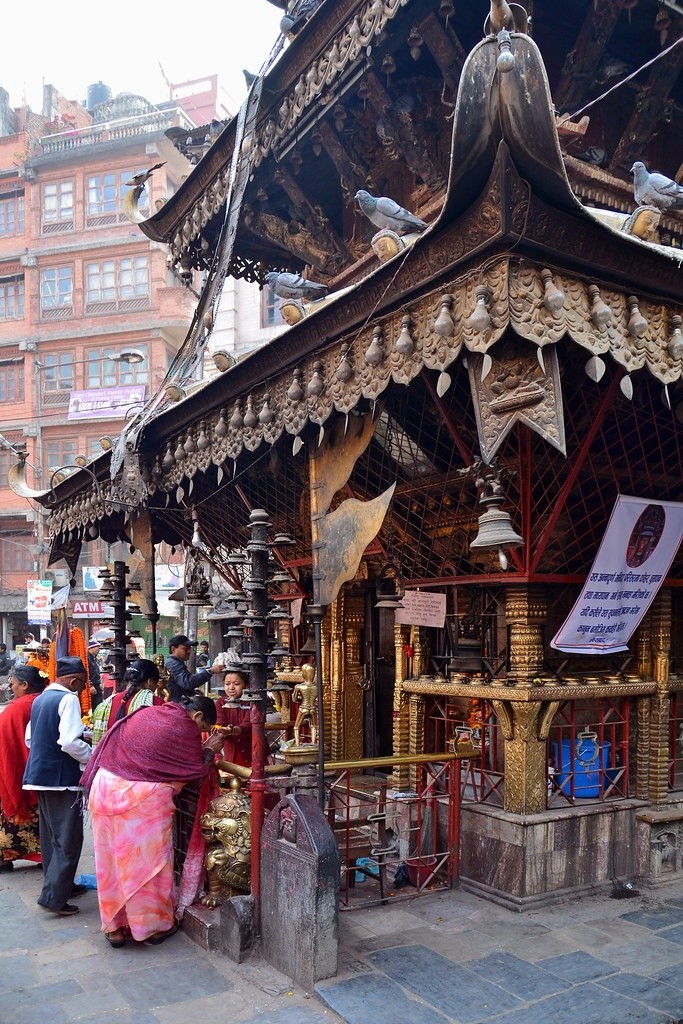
630, 161, 683, 213
354, 190, 428, 232
261, 272, 329, 303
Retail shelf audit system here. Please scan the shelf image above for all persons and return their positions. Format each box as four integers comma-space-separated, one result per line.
164, 635, 212, 703
207, 669, 266, 778
292, 663, 318, 746
79, 696, 225, 947
86, 640, 102, 713
0, 666, 48, 871
87, 659, 166, 760
0, 632, 52, 675
22, 657, 91, 916
153, 652, 168, 692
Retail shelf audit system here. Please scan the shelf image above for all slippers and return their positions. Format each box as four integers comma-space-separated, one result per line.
148, 926, 179, 945
105, 932, 126, 948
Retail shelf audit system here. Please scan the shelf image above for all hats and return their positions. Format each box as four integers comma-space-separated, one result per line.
170, 635, 199, 647
56, 656, 87, 678
88, 641, 101, 649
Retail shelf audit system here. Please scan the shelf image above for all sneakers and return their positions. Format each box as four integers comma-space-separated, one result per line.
69, 883, 88, 897
37, 902, 79, 915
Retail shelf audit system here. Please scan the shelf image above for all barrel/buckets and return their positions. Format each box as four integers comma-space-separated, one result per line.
553, 739, 620, 798
547, 766, 555, 796
404, 855, 437, 887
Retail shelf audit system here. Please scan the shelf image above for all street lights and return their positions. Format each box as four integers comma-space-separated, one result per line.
34, 346, 146, 643
